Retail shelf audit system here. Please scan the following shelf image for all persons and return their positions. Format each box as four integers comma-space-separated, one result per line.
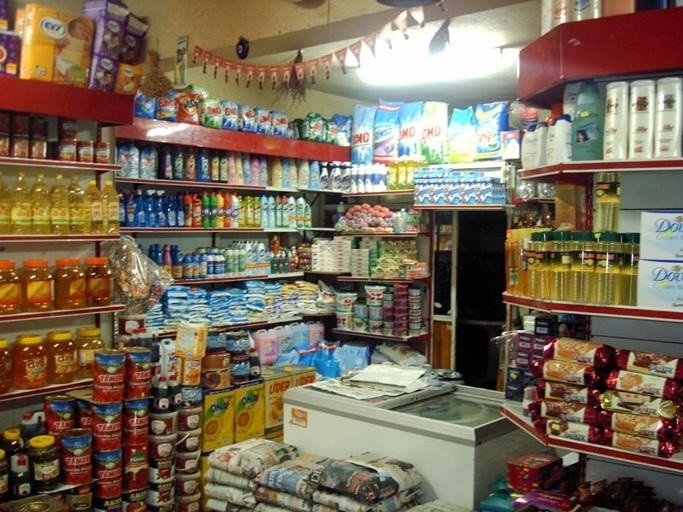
575, 130, 590, 144
53, 15, 93, 77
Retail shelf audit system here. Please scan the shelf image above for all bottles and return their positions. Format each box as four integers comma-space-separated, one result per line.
336, 201, 345, 222
504, 227, 638, 305
248, 348, 262, 379
571, 85, 604, 160
350, 155, 429, 191
0, 173, 119, 234
150, 365, 184, 412
593, 173, 620, 233
147, 240, 271, 279
392, 207, 407, 231
161, 145, 320, 188
168, 188, 311, 229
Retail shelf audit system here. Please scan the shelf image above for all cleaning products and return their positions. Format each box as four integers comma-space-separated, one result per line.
115, 189, 313, 281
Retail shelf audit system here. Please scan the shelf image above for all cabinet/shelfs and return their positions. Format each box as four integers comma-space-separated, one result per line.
0, 4, 683, 511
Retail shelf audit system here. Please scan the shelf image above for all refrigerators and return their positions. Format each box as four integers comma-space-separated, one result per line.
281, 370, 556, 511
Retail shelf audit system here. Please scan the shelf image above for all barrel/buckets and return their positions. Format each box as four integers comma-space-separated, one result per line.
252, 321, 325, 364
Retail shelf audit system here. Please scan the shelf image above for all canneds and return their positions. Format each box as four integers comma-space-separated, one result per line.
1, 110, 110, 164
0, 257, 151, 512
